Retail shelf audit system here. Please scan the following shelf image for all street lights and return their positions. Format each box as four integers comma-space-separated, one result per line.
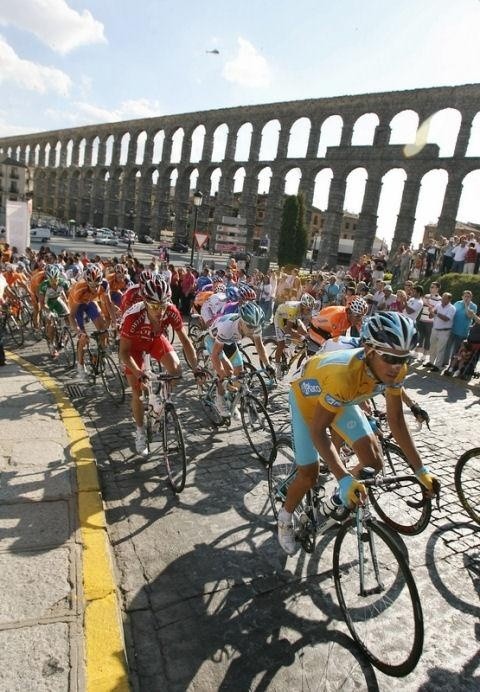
189, 188, 203, 267
309, 230, 319, 273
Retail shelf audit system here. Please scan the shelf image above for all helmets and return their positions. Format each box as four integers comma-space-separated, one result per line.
300, 292, 315, 308
138, 270, 156, 282
345, 295, 369, 315
113, 263, 129, 279
43, 263, 60, 280
359, 310, 419, 351
140, 278, 176, 303
240, 301, 264, 328
196, 275, 258, 301
1, 258, 47, 272
83, 265, 103, 287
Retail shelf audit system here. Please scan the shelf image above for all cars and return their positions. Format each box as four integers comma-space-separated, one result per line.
30, 212, 153, 246
168, 242, 188, 253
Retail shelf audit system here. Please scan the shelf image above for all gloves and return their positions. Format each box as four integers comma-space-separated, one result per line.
337, 472, 368, 510
265, 364, 277, 381
221, 377, 239, 393
414, 466, 441, 495
411, 401, 432, 424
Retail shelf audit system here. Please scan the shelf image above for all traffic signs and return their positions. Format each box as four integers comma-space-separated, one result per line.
159, 236, 175, 241
161, 231, 174, 236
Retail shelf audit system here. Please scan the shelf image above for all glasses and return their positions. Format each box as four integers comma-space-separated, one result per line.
246, 323, 259, 330
373, 349, 413, 366
145, 300, 169, 311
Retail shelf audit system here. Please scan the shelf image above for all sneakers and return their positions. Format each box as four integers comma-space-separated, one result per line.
77, 362, 88, 378
276, 507, 297, 556
49, 348, 60, 361
133, 428, 151, 457
32, 326, 68, 360
214, 396, 232, 418
411, 354, 461, 379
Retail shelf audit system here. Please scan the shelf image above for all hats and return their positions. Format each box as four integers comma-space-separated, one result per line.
383, 285, 394, 293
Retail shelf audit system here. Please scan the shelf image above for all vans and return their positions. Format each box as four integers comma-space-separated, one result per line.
0, 226, 6, 240
